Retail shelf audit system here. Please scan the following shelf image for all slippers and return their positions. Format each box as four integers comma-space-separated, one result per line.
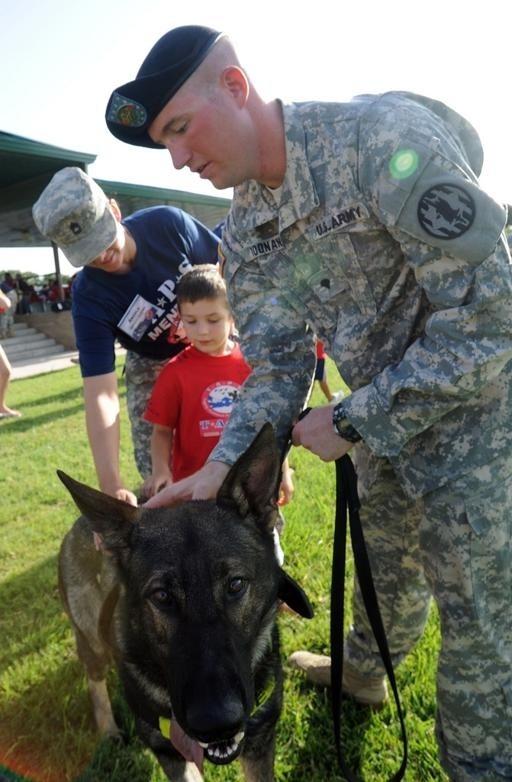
0, 410, 20, 418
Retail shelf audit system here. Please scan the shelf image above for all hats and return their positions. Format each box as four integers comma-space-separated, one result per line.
105, 26, 226, 149
31, 166, 119, 269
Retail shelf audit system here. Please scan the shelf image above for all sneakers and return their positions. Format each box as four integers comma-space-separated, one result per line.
287, 650, 389, 706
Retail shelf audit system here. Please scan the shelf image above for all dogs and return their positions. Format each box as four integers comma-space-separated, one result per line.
57, 421, 285, 782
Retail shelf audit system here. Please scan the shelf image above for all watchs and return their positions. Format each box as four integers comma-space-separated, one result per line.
333, 402, 363, 445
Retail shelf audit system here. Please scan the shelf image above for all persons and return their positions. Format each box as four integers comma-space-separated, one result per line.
141, 259, 295, 507
313, 339, 343, 404
0, 271, 74, 338
34, 165, 221, 509
0, 289, 20, 418
106, 25, 512, 780
